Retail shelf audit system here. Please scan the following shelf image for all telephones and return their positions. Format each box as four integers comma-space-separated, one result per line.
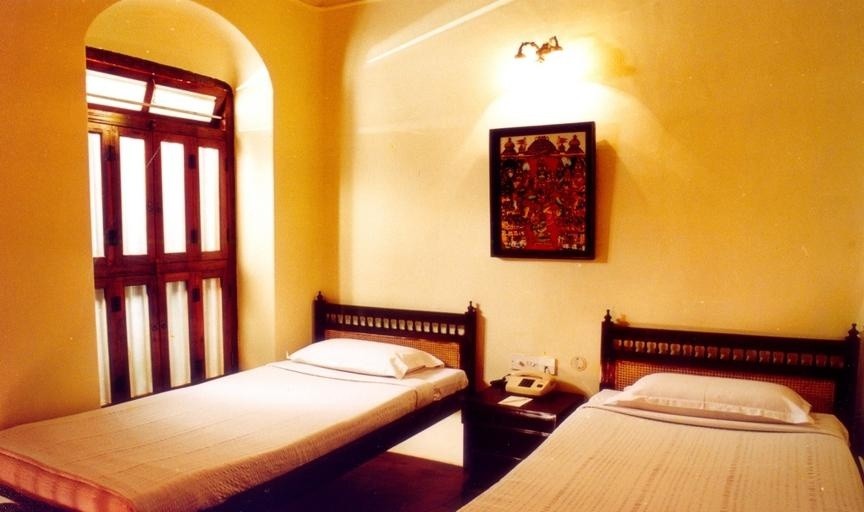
505, 369, 557, 398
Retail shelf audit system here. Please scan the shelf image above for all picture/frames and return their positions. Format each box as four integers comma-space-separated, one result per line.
489, 121, 596, 260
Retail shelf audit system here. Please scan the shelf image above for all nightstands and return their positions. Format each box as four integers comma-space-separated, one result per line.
466, 380, 586, 481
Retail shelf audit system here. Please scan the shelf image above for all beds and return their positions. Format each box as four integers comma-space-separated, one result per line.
0, 289, 497, 512
448, 310, 863, 512
